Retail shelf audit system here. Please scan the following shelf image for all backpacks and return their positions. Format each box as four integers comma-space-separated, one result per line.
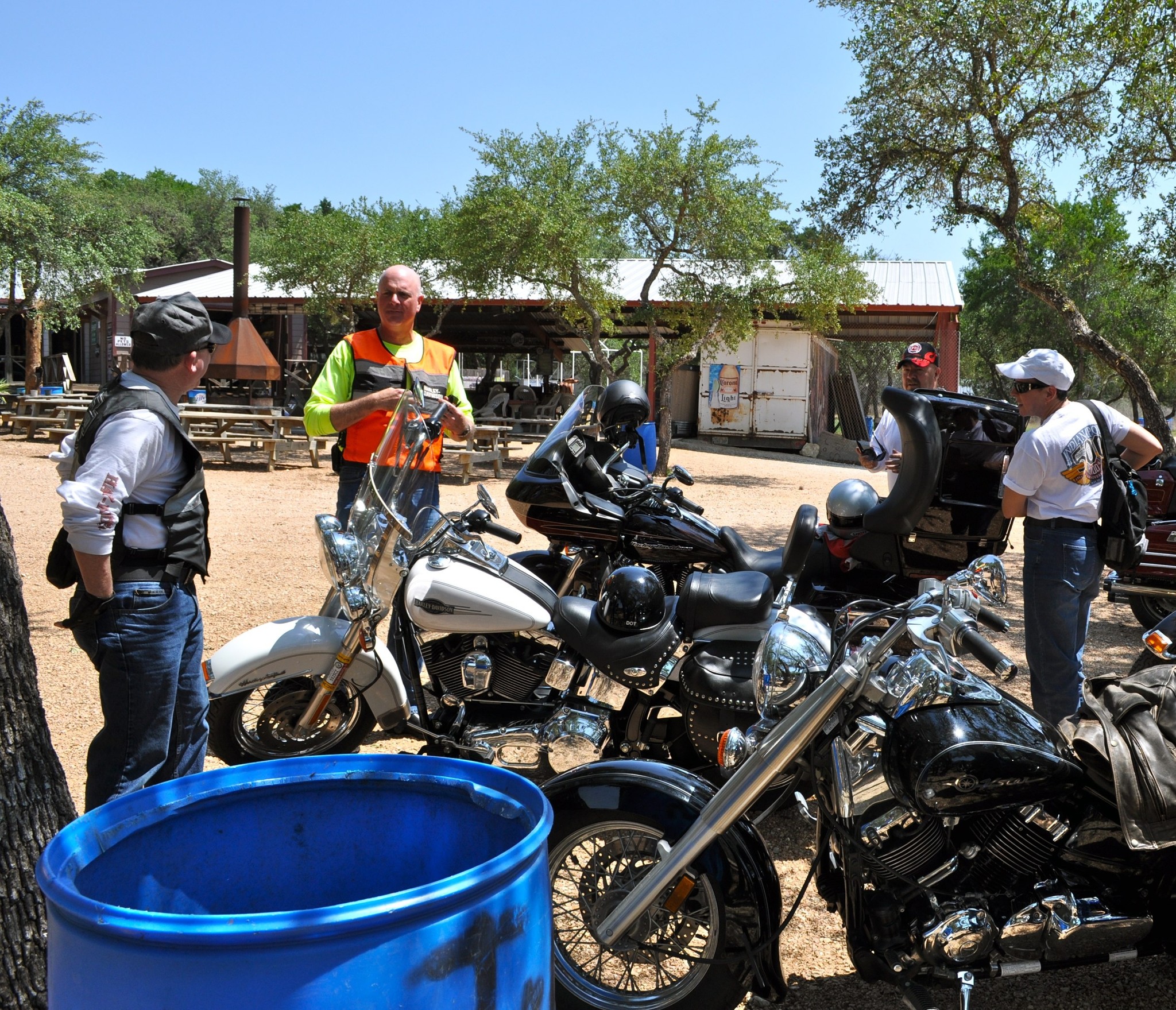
1077, 399, 1149, 575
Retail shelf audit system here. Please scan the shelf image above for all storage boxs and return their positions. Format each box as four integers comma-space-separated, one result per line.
1135, 470, 1175, 515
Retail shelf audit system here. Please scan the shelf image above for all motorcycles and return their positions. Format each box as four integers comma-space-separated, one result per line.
506, 384, 1026, 635
201, 377, 918, 811
1103, 453, 1176, 677
537, 554, 1176, 1010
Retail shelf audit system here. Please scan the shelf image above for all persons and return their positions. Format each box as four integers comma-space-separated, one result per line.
301, 263, 476, 714
995, 349, 1163, 729
856, 342, 941, 494
48, 291, 233, 815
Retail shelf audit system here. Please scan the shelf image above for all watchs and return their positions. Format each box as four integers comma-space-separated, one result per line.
456, 425, 471, 437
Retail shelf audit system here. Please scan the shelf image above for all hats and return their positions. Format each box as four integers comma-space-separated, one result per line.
995, 349, 1075, 391
897, 342, 939, 370
130, 292, 233, 357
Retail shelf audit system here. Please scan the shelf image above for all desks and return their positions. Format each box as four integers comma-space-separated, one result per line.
56, 406, 89, 428
25, 398, 93, 438
178, 409, 320, 471
500, 400, 539, 433
458, 424, 513, 483
11, 392, 87, 434
473, 416, 559, 461
176, 401, 285, 448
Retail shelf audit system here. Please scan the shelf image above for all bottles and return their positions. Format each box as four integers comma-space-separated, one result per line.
718, 365, 739, 408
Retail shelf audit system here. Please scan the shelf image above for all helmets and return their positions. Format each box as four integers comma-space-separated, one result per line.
597, 379, 650, 440
825, 479, 878, 537
597, 566, 667, 638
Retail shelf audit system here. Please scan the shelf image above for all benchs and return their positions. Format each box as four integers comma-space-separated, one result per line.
177, 422, 339, 452
439, 434, 547, 463
1, 409, 84, 442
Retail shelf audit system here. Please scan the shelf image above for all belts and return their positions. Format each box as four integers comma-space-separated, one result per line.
1024, 516, 1098, 530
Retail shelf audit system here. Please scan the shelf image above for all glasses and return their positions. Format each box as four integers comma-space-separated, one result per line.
1013, 378, 1045, 394
184, 341, 216, 354
854, 431, 886, 461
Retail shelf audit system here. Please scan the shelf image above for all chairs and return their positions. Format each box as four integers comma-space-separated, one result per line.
474, 384, 582, 434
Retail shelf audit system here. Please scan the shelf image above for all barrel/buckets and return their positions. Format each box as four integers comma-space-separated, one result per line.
16, 387, 63, 394
37, 753, 553, 1010
621, 422, 657, 474
866, 417, 873, 439
